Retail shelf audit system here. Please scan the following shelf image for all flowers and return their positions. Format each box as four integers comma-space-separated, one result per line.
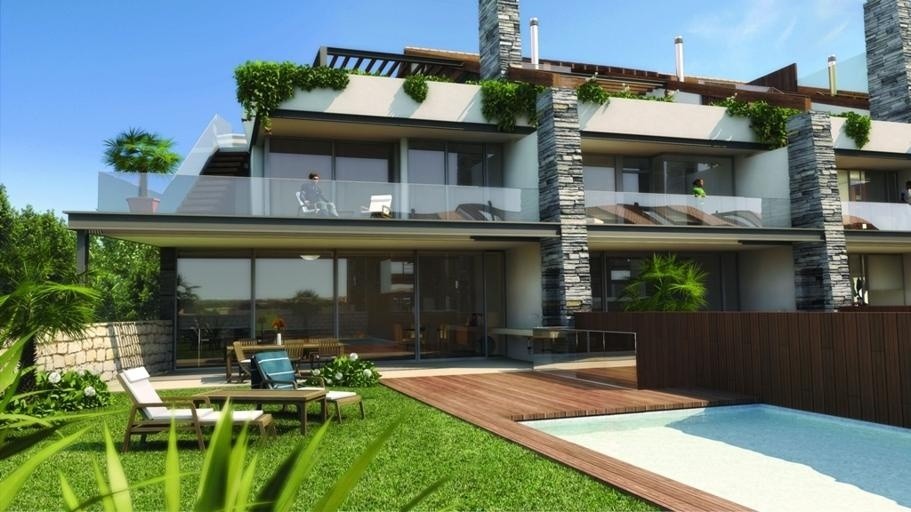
271, 318, 286, 333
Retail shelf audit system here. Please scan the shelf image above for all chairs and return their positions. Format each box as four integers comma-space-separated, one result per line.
295, 192, 321, 218
115, 335, 365, 453
360, 194, 393, 219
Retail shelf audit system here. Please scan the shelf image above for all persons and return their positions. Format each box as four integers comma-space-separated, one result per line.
901, 180, 911, 204
693, 178, 708, 198
299, 171, 340, 218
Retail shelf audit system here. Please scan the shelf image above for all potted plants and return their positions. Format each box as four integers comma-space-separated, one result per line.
100, 126, 185, 215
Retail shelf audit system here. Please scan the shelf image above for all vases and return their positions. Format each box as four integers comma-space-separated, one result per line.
276, 331, 282, 345
